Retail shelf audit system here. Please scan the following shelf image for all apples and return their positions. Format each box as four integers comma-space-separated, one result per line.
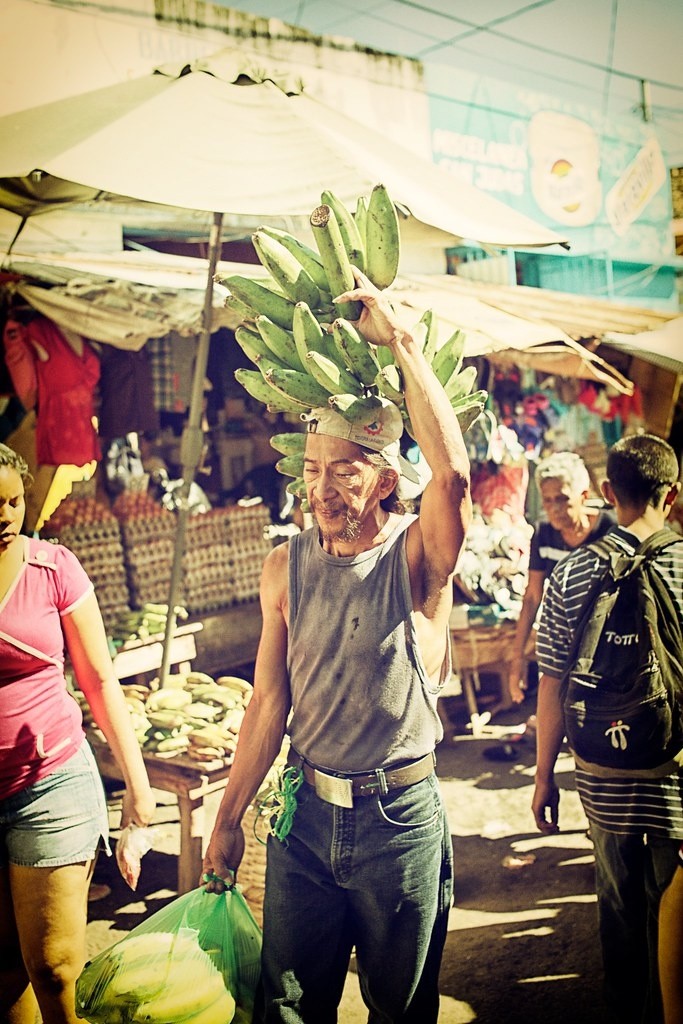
41, 491, 273, 629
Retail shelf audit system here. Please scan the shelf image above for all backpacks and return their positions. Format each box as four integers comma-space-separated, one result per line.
558, 527, 683, 771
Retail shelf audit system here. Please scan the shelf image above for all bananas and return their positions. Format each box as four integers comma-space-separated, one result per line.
64, 599, 252, 760
74, 926, 260, 1024
212, 185, 488, 511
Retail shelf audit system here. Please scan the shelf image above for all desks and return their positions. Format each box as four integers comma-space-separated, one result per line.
70, 633, 196, 690
442, 621, 538, 725
81, 733, 290, 895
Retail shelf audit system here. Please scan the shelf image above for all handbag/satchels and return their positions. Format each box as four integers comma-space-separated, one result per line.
75, 870, 263, 1024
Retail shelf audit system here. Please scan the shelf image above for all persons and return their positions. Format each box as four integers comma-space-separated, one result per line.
201, 264, 470, 1024
0, 444, 155, 1024
509, 433, 683, 1024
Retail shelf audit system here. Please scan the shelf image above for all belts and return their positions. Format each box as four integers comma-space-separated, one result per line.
287, 743, 436, 809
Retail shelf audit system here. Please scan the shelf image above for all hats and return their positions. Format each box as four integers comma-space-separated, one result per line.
300, 396, 402, 475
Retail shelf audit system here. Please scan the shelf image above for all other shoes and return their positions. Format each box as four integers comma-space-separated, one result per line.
482, 745, 520, 762
